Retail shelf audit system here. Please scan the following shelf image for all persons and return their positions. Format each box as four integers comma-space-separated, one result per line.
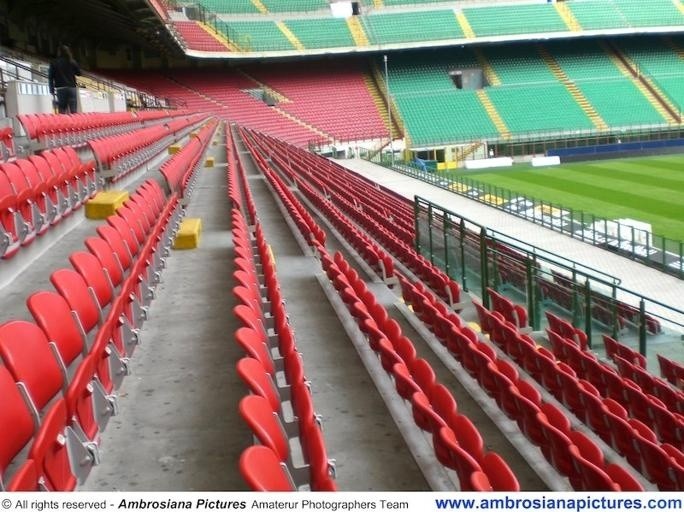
49, 45, 81, 114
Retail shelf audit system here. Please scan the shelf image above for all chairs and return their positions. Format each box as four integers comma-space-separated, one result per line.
601, 334, 616, 359
671, 457, 684, 491
50, 269, 127, 353
208, 20, 297, 52
158, 116, 221, 199
502, 324, 535, 369
469, 471, 493, 491
0, 163, 49, 236
0, 321, 99, 441
617, 343, 647, 368
486, 363, 541, 422
565, 445, 644, 492
382, 0, 457, 7
116, 207, 162, 249
26, 114, 57, 149
27, 290, 115, 393
69, 251, 136, 327
508, 386, 571, 446
483, 82, 596, 135
170, 21, 232, 52
16, 159, 61, 226
648, 400, 683, 440
566, 0, 684, 30
461, 3, 570, 37
136, 188, 173, 217
0, 190, 20, 260
622, 382, 666, 427
555, 369, 600, 422
562, 339, 597, 373
622, 47, 684, 114
95, 70, 332, 148
536, 413, 604, 475
468, 344, 518, 382
358, 9, 466, 45
63, 109, 200, 146
657, 355, 674, 385
362, 0, 375, 6
123, 200, 166, 232
96, 224, 151, 288
393, 363, 457, 415
235, 358, 314, 440
35, 112, 63, 146
487, 53, 559, 85
679, 0, 684, 6
531, 350, 576, 403
676, 419, 684, 447
16, 114, 48, 148
519, 339, 555, 385
40, 151, 82, 210
259, 1, 330, 13
7, 458, 55, 493
378, 338, 436, 385
552, 52, 668, 128
85, 236, 143, 304
282, 17, 357, 49
234, 327, 305, 408
600, 367, 641, 404
28, 154, 72, 218
413, 393, 483, 461
582, 355, 617, 389
578, 389, 628, 446
106, 215, 157, 269
434, 427, 520, 492
86, 114, 211, 184
369, 329, 416, 360
633, 430, 684, 491
379, 58, 501, 146
43, 113, 70, 145
239, 61, 389, 141
129, 193, 170, 223
0, 170, 36, 247
193, 0, 261, 14
61, 146, 97, 198
602, 406, 657, 473
0, 365, 77, 493
224, 122, 669, 333
0, 128, 15, 157
543, 328, 580, 359
447, 327, 496, 360
672, 362, 684, 389
239, 445, 336, 492
51, 148, 90, 204
238, 396, 328, 491
